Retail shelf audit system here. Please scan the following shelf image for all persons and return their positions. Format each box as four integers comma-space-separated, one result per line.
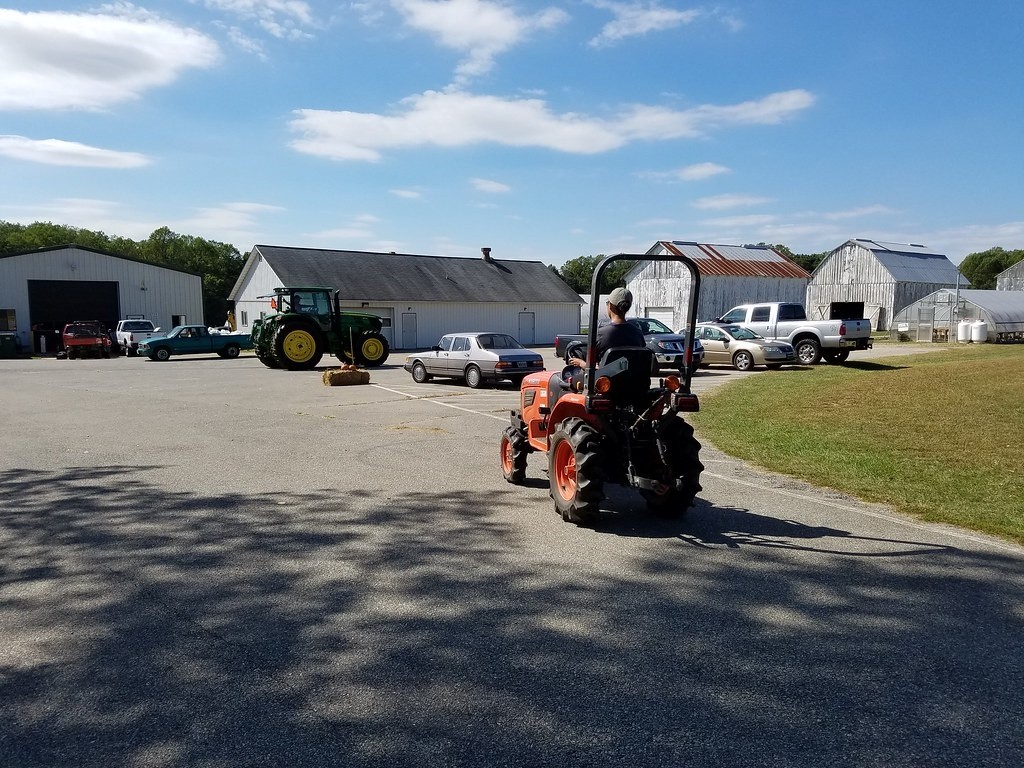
569, 288, 646, 370
289, 295, 302, 312
227, 311, 236, 331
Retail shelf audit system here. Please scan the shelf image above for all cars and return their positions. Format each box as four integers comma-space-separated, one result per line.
672, 322, 796, 370
403, 331, 546, 388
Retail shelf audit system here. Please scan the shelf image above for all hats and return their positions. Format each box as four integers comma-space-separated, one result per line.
608, 288, 633, 307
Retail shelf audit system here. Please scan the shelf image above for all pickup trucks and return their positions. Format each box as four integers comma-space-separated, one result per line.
116, 319, 167, 357
555, 318, 704, 376
716, 301, 874, 364
136, 325, 255, 361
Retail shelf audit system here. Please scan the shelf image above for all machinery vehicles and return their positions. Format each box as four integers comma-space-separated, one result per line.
251, 286, 389, 370
499, 255, 705, 522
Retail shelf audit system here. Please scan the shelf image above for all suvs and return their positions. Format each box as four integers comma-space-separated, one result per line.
63, 320, 112, 360
